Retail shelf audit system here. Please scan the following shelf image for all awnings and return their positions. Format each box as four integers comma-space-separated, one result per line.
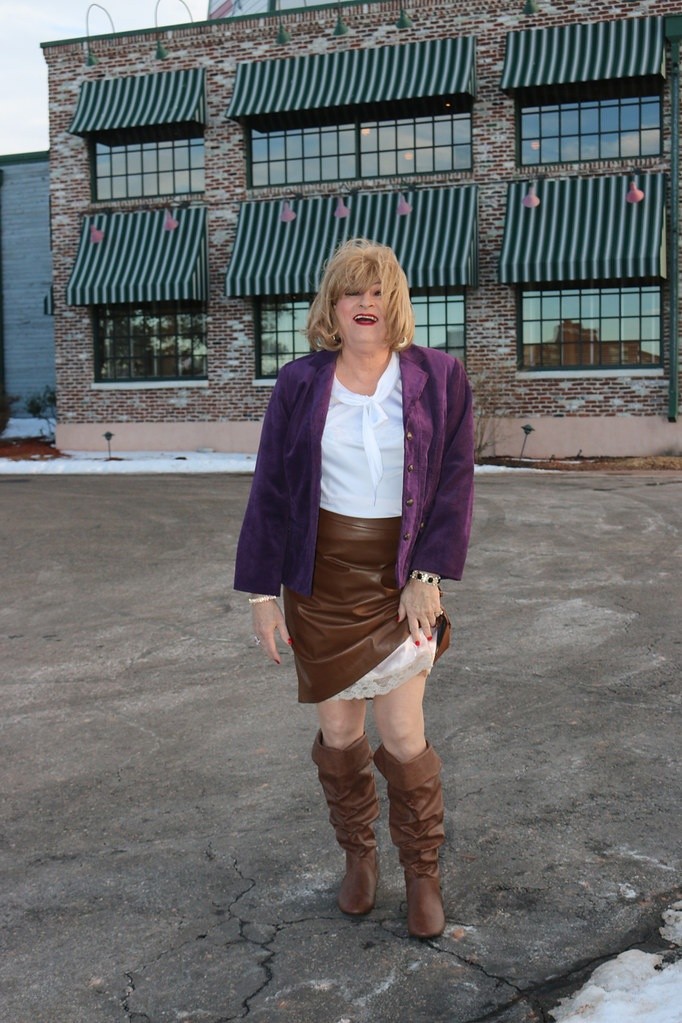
499, 15, 666, 99
225, 35, 478, 127
66, 208, 209, 307
68, 67, 207, 139
225, 185, 478, 297
497, 172, 668, 283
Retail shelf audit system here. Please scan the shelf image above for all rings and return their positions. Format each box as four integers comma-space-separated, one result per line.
254, 636, 260, 645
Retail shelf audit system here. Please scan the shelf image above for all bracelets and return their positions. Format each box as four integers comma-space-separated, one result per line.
249, 595, 276, 604
410, 571, 441, 586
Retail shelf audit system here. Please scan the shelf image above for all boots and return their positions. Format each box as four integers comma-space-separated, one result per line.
311, 729, 379, 915
374, 734, 447, 938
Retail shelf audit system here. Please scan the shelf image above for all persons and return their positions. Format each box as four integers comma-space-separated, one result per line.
233, 239, 475, 940
